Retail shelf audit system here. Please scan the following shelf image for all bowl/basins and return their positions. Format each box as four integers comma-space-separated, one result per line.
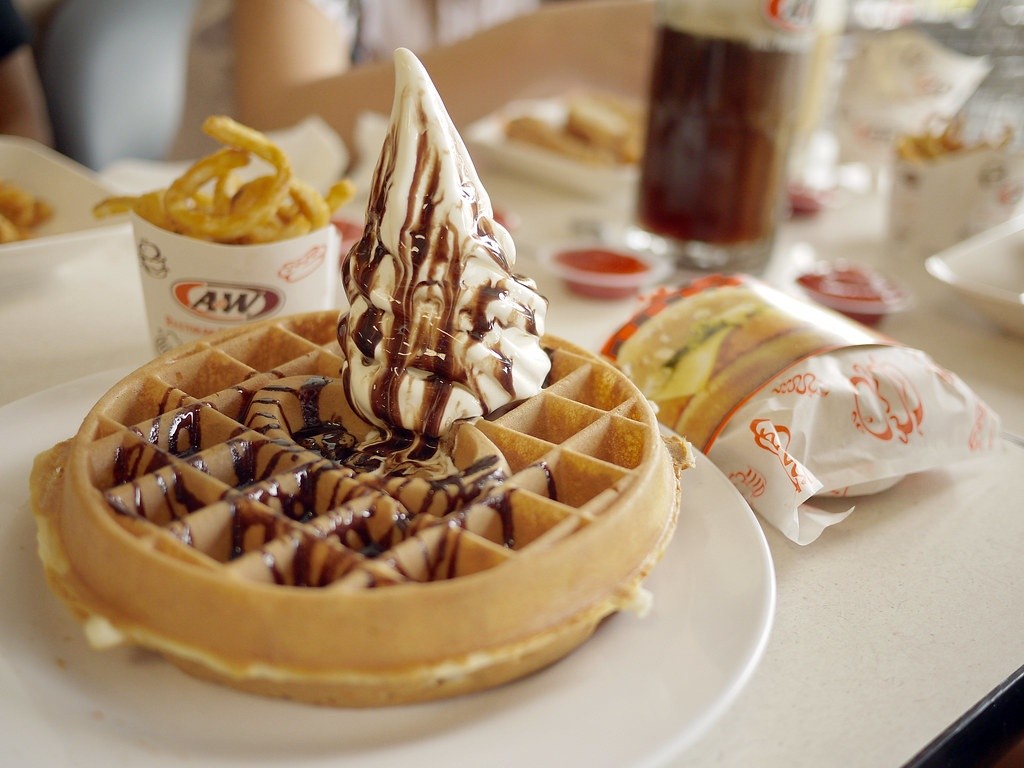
792, 263, 913, 327
535, 237, 673, 306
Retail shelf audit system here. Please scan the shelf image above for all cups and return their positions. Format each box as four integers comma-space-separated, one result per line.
625, 0, 821, 274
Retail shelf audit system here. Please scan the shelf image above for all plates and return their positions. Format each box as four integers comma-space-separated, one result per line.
0, 134, 137, 290
924, 212, 1024, 336
0, 360, 776, 768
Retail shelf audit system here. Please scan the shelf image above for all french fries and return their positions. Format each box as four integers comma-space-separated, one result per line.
97, 115, 359, 246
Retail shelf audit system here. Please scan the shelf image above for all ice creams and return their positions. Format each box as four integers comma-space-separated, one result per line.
342, 47, 552, 431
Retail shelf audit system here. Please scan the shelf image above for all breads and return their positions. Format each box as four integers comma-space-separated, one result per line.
29, 311, 695, 704
